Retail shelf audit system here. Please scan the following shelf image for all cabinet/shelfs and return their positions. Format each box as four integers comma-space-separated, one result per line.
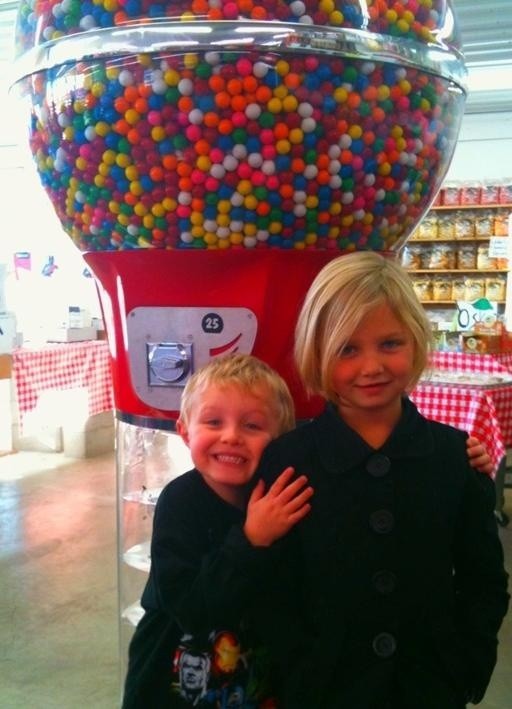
400, 202, 512, 308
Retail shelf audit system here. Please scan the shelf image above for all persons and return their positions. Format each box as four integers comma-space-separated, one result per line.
172, 649, 211, 707
122, 355, 495, 709
243, 250, 510, 709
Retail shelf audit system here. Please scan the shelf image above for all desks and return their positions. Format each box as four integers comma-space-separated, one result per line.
406, 341, 512, 514
